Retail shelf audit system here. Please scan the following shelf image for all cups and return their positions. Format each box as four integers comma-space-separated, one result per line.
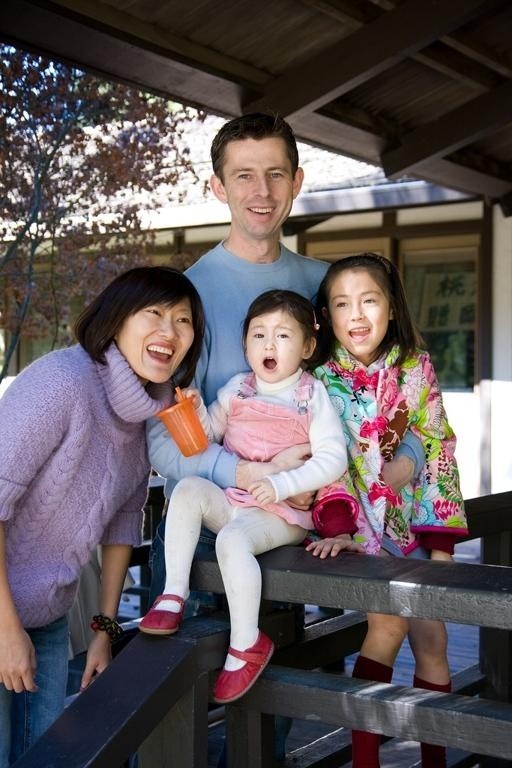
156, 395, 211, 458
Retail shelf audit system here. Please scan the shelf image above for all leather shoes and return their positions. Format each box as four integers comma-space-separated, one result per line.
138, 594, 185, 635
212, 630, 276, 704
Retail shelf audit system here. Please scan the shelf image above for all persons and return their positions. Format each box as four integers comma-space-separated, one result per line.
138, 290, 348, 704
0, 267, 206, 767
304, 254, 470, 768
147, 111, 331, 768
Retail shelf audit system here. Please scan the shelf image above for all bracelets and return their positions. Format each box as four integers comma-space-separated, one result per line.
91, 615, 124, 645
